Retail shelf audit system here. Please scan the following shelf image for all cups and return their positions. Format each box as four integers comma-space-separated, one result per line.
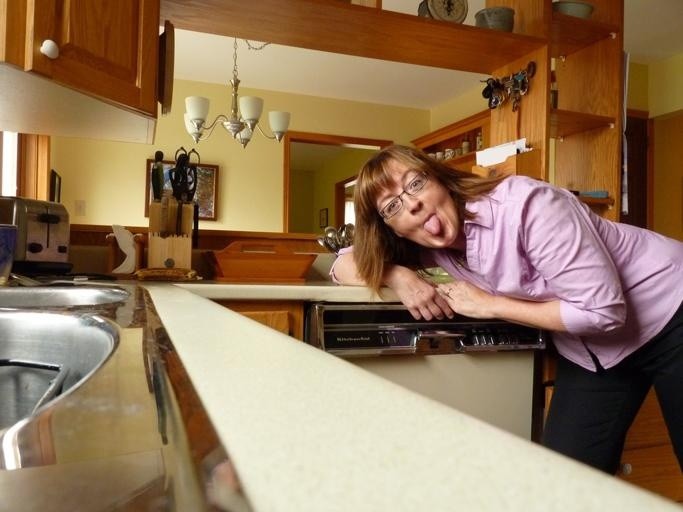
1, 223, 16, 285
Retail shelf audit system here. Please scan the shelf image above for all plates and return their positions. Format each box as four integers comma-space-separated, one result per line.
418, 266, 456, 286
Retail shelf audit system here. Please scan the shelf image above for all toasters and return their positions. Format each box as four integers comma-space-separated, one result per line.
1, 195, 71, 268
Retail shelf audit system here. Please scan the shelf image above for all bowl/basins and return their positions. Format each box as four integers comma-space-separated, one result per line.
552, 2, 593, 20
474, 6, 516, 33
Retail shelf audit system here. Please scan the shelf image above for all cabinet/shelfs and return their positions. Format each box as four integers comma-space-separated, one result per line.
481, 0, 623, 223
0, 0, 161, 123
542, 343, 683, 503
215, 298, 307, 346
410, 111, 493, 167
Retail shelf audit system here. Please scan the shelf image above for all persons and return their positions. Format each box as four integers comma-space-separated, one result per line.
327, 141, 682, 488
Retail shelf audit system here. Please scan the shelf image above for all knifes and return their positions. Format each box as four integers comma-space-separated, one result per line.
151, 148, 165, 200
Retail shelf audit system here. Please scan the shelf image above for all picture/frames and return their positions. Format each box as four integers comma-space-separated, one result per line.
319, 207, 328, 228
144, 157, 220, 222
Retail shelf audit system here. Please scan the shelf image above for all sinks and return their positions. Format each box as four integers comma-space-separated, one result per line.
0, 309, 120, 438
0, 282, 130, 308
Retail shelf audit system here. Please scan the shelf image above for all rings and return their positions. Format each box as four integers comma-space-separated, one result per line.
444, 288, 452, 297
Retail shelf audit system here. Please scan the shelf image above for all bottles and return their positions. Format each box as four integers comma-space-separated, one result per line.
424, 133, 482, 160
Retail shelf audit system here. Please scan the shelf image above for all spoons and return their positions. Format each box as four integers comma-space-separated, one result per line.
318, 223, 356, 252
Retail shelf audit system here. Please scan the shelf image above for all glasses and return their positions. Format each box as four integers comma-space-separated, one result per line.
378, 170, 431, 220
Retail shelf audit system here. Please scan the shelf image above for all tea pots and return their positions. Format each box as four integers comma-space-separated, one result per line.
328, 244, 370, 287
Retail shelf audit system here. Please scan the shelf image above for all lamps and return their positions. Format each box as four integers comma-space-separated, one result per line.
181, 36, 293, 150
